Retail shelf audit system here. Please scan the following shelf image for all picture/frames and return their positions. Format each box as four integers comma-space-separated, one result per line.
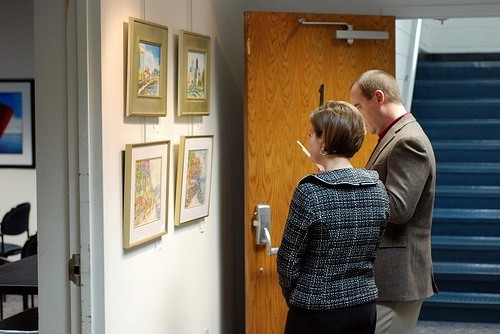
126, 16, 168, 118
174, 135, 213, 227
177, 29, 212, 117
123, 140, 172, 251
0, 78, 38, 170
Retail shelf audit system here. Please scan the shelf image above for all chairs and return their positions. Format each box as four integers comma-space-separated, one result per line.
0, 202, 33, 303
20, 234, 38, 308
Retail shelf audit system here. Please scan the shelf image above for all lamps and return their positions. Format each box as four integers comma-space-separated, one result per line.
298, 19, 389, 44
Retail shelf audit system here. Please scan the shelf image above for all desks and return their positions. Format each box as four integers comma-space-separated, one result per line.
0, 255, 38, 321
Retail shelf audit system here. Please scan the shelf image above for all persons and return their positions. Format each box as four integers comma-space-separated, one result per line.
349, 70, 440, 334
276, 100, 391, 334
